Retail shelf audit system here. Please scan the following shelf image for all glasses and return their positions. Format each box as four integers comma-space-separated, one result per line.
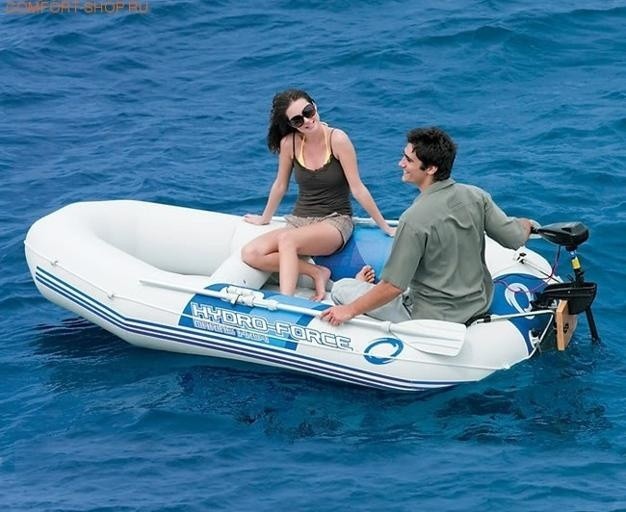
288, 104, 316, 129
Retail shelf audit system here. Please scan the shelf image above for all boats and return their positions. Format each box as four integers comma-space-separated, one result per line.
24, 198, 601, 396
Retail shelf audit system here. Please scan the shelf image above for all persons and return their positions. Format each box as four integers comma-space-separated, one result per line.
240, 88, 397, 303
318, 125, 536, 326
